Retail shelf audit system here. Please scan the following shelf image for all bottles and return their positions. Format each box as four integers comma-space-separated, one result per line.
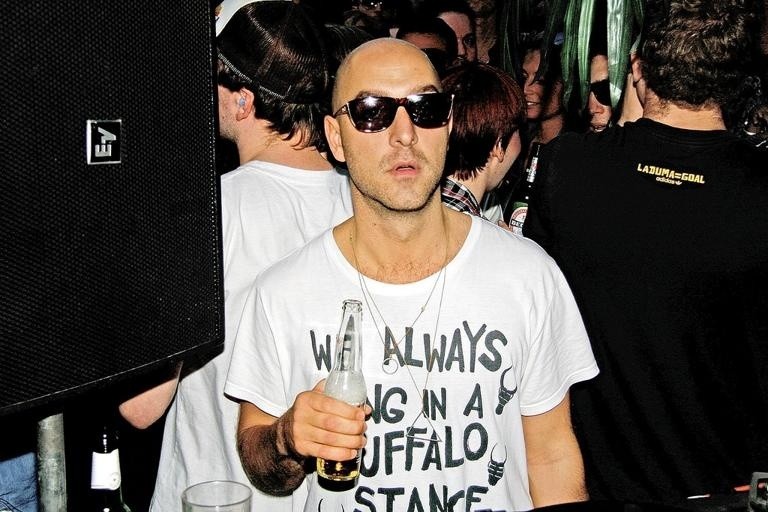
316, 299, 367, 492
84, 422, 132, 510
506, 144, 541, 233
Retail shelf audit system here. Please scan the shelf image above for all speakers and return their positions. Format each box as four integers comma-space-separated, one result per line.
1, 0, 225, 431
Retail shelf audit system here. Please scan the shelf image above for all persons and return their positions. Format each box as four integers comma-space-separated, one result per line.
482, 44, 566, 219
521, 0, 768, 512
396, 17, 458, 79
430, 0, 476, 61
442, 62, 528, 220
225, 37, 600, 512
117, 0, 352, 512
586, 50, 612, 133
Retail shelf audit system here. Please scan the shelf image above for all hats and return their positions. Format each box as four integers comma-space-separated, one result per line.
216, 0, 330, 104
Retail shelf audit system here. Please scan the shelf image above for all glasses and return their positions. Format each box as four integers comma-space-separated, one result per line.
333, 92, 455, 134
591, 79, 612, 107
360, 0, 384, 10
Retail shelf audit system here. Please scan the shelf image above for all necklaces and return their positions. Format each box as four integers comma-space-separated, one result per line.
350, 209, 449, 374
356, 258, 449, 442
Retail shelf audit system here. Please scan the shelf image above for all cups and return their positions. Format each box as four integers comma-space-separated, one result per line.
179, 479, 253, 511
